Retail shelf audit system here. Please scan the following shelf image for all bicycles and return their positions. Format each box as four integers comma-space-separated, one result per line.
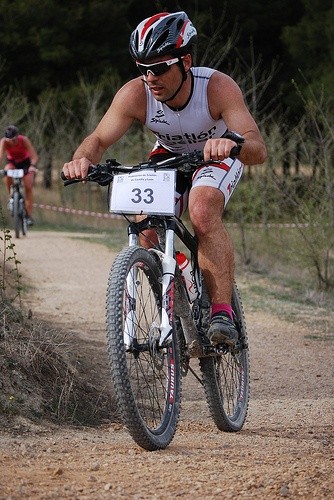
1, 168, 33, 238
61, 146, 251, 453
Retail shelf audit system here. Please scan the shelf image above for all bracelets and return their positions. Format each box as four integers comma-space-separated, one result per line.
29, 163, 35, 167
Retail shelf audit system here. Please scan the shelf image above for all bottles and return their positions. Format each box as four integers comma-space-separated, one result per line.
175, 250, 198, 303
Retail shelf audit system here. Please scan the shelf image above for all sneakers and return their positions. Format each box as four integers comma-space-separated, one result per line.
207, 310, 238, 345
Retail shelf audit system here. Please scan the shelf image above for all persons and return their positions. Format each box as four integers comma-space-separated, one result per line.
62, 12, 267, 350
0, 125, 38, 225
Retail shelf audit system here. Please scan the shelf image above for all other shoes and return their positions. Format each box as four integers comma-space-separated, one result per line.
8, 200, 13, 209
28, 217, 33, 225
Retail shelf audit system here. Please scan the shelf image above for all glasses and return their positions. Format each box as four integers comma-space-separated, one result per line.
136, 55, 185, 77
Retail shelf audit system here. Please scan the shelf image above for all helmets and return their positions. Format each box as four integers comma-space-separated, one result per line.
129, 11, 197, 61
5, 126, 17, 141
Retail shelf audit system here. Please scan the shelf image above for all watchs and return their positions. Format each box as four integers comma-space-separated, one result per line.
223, 130, 246, 156
